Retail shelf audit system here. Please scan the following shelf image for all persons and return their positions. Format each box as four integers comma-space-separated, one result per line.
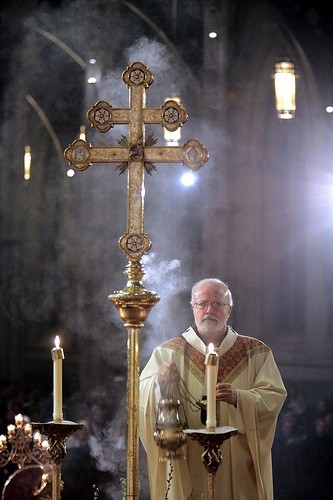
0, 392, 333, 500
141, 278, 287, 500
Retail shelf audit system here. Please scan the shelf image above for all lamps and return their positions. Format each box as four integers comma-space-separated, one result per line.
274, 28, 296, 122
0, 414, 49, 470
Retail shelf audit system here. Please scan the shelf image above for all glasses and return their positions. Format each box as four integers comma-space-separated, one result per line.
194, 301, 230, 310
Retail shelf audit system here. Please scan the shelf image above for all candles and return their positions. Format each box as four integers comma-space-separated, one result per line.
204, 343, 219, 431
52, 334, 63, 421
